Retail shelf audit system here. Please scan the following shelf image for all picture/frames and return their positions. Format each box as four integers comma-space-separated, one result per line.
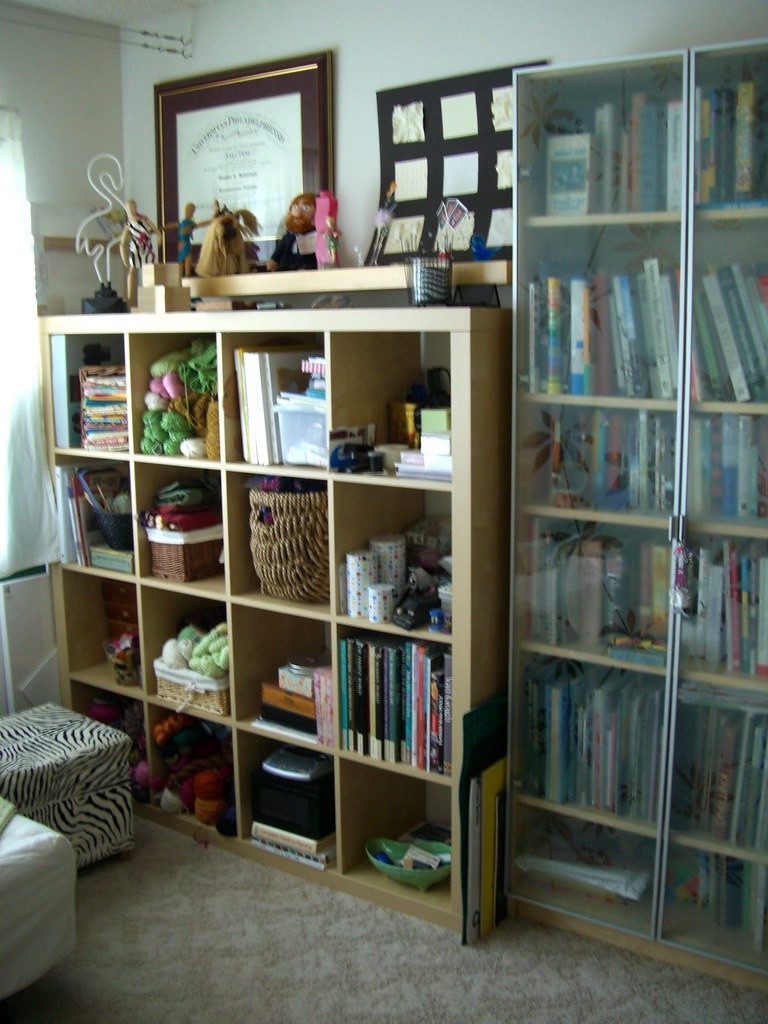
149, 49, 335, 271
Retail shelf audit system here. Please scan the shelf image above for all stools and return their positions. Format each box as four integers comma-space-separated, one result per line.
0, 702, 136, 872
0, 814, 77, 1001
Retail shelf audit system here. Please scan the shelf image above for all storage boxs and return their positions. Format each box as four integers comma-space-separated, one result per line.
424, 455, 452, 473
277, 411, 327, 467
420, 409, 451, 432
102, 581, 139, 640
259, 703, 317, 734
261, 678, 316, 717
420, 435, 451, 455
390, 402, 418, 446
250, 768, 334, 840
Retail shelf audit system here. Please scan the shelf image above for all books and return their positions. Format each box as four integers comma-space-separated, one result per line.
672, 538, 768, 951
395, 451, 454, 483
251, 820, 336, 871
53, 460, 121, 566
521, 658, 664, 825
314, 667, 334, 747
234, 346, 323, 467
686, 79, 768, 526
527, 257, 681, 400
546, 408, 676, 515
526, 518, 670, 668
338, 633, 453, 776
545, 90, 681, 216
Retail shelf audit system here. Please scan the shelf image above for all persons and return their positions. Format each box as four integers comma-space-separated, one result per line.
323, 216, 341, 269
438, 200, 464, 229
568, 163, 585, 191
266, 192, 323, 271
163, 204, 212, 278
120, 199, 161, 303
367, 181, 398, 266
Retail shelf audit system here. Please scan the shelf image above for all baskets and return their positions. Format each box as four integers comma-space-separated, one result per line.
248, 488, 330, 603
145, 527, 224, 584
95, 511, 134, 551
153, 657, 230, 717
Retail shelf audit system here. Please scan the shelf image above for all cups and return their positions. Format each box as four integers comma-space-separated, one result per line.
411, 256, 452, 307
347, 531, 406, 624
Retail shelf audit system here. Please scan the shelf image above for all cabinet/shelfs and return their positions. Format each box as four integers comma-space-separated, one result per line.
37, 307, 509, 940
496, 35, 768, 992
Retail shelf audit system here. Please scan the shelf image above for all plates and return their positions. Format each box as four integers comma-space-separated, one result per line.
365, 841, 452, 892
